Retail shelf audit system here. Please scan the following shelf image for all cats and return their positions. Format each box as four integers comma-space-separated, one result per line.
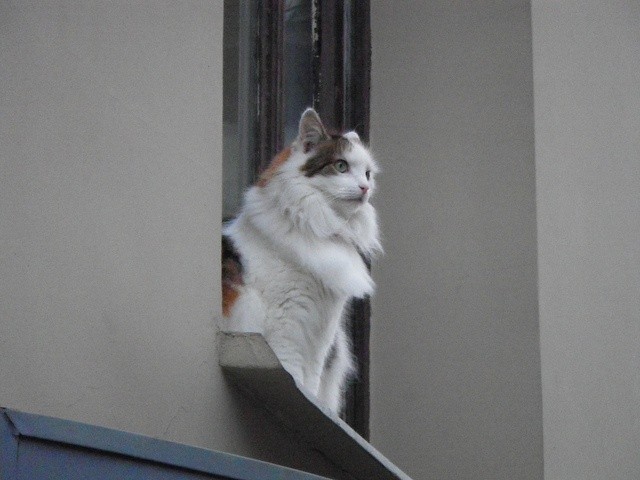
223, 106, 386, 412
